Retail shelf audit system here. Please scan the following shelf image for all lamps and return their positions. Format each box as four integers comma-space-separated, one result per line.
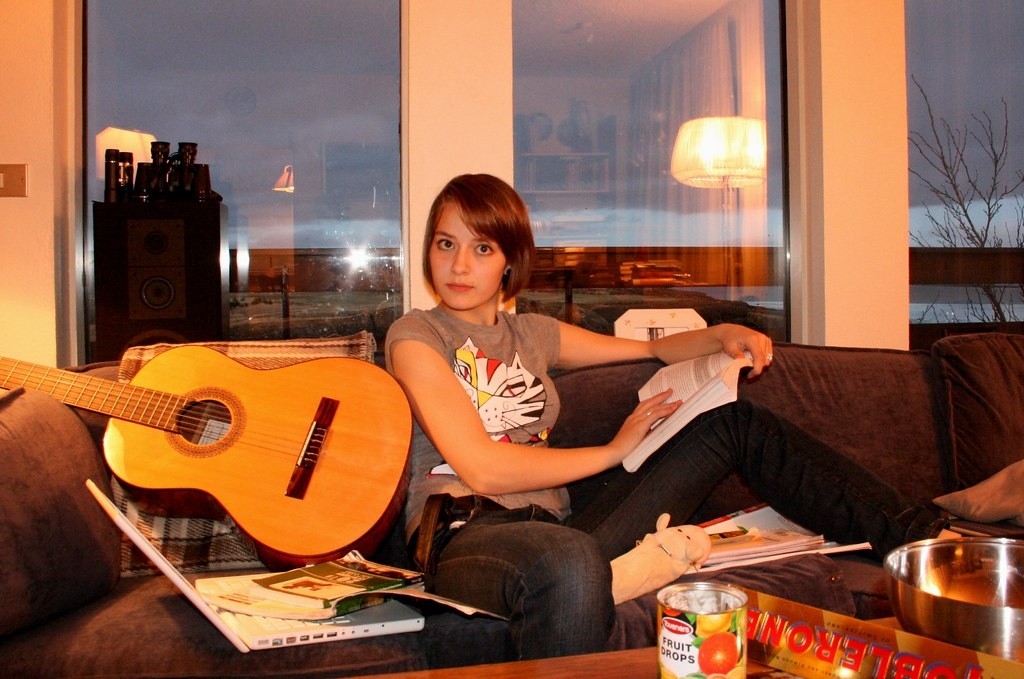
670, 116, 767, 302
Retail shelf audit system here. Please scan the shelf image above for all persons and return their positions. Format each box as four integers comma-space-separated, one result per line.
385, 174, 961, 661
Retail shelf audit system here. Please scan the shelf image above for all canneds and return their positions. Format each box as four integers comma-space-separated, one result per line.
657, 581, 748, 679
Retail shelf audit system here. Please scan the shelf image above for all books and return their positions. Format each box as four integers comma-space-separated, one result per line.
622, 350, 753, 473
636, 503, 872, 575
194, 550, 509, 651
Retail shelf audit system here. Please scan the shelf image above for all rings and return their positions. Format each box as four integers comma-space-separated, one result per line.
765, 353, 773, 360
647, 412, 651, 415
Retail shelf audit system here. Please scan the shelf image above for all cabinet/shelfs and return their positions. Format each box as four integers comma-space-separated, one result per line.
515, 151, 610, 194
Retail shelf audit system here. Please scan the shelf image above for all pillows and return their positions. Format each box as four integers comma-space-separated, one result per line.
931, 461, 1023, 528
933, 333, 1024, 488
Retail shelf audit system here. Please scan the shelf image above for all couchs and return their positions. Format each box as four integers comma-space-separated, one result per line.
0, 331, 1024, 679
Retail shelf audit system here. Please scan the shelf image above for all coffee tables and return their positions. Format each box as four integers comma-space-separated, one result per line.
352, 615, 906, 679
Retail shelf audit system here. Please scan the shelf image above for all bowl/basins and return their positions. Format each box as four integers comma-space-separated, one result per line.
885, 537, 1024, 665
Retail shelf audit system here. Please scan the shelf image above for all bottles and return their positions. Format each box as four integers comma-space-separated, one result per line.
104, 142, 213, 202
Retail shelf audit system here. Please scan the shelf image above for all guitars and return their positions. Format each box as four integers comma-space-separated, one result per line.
0, 344, 414, 570
